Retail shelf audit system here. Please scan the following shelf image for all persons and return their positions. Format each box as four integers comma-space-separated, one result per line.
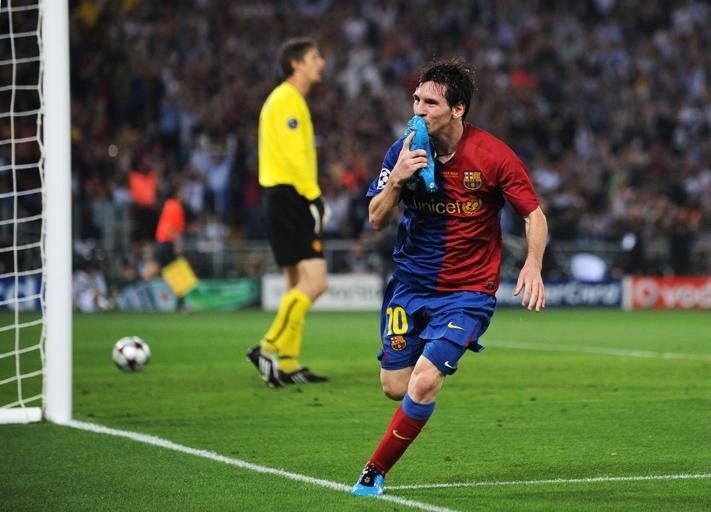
351, 62, 548, 499
244, 38, 332, 389
1, 2, 711, 313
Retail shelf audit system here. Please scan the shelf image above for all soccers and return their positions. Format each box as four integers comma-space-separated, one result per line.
112, 336, 151, 372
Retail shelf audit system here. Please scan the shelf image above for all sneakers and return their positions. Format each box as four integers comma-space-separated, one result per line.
279, 368, 328, 384
247, 347, 283, 388
351, 464, 384, 497
